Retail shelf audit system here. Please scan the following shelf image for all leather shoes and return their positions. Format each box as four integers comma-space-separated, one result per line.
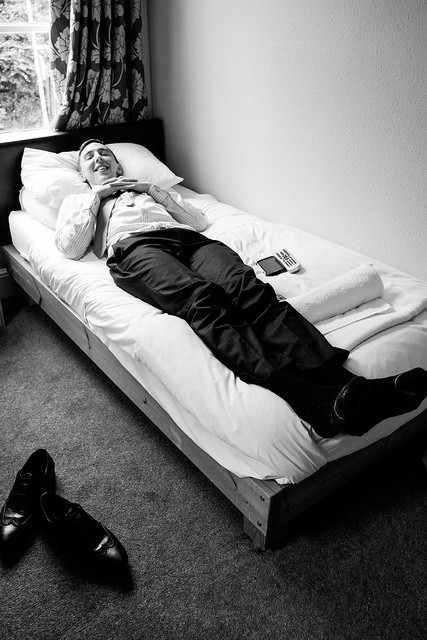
38, 491, 128, 572
1, 449, 56, 570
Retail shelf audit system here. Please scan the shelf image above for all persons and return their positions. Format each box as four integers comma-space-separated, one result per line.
56, 139, 427, 437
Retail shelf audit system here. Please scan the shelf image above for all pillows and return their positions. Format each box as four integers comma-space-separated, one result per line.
14, 185, 186, 230
20, 142, 188, 207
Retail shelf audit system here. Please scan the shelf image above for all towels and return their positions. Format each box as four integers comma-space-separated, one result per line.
280, 264, 392, 336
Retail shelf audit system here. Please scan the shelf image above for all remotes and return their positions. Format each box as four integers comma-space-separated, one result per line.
273, 248, 300, 273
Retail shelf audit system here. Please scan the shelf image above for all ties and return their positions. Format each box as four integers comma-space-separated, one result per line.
92, 190, 134, 259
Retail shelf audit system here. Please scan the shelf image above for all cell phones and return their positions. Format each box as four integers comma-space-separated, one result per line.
256, 255, 286, 277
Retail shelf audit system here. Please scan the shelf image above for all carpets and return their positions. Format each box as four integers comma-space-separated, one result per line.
0, 297, 427, 640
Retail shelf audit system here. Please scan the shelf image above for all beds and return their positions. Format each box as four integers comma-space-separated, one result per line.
0, 114, 426, 550
